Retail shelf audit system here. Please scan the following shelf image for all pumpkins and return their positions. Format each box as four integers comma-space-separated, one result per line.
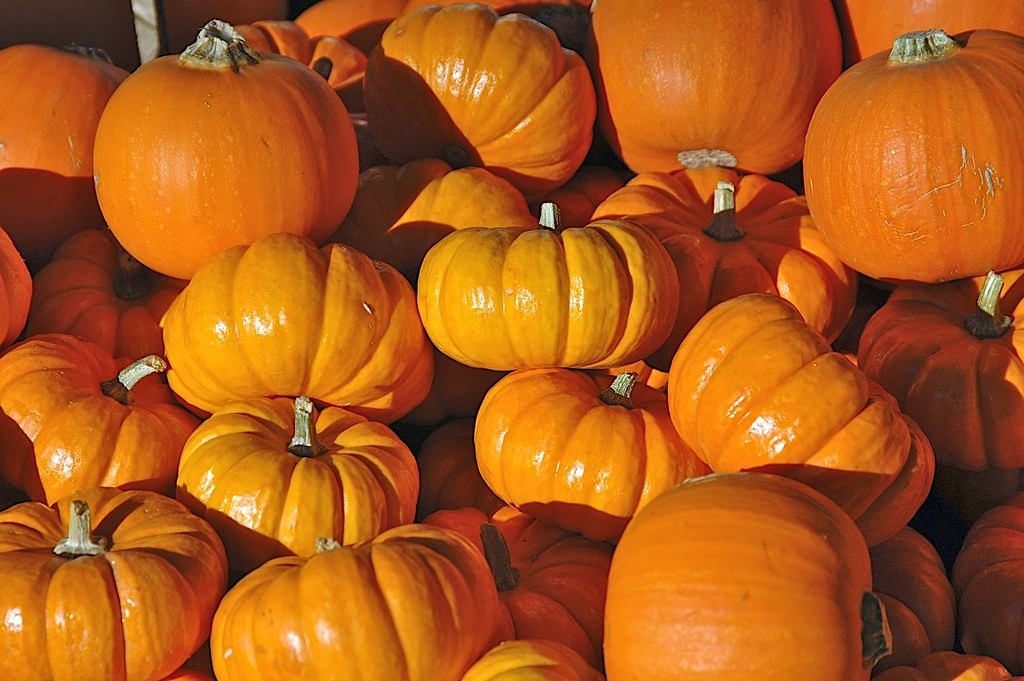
0, 0, 1024, 681
